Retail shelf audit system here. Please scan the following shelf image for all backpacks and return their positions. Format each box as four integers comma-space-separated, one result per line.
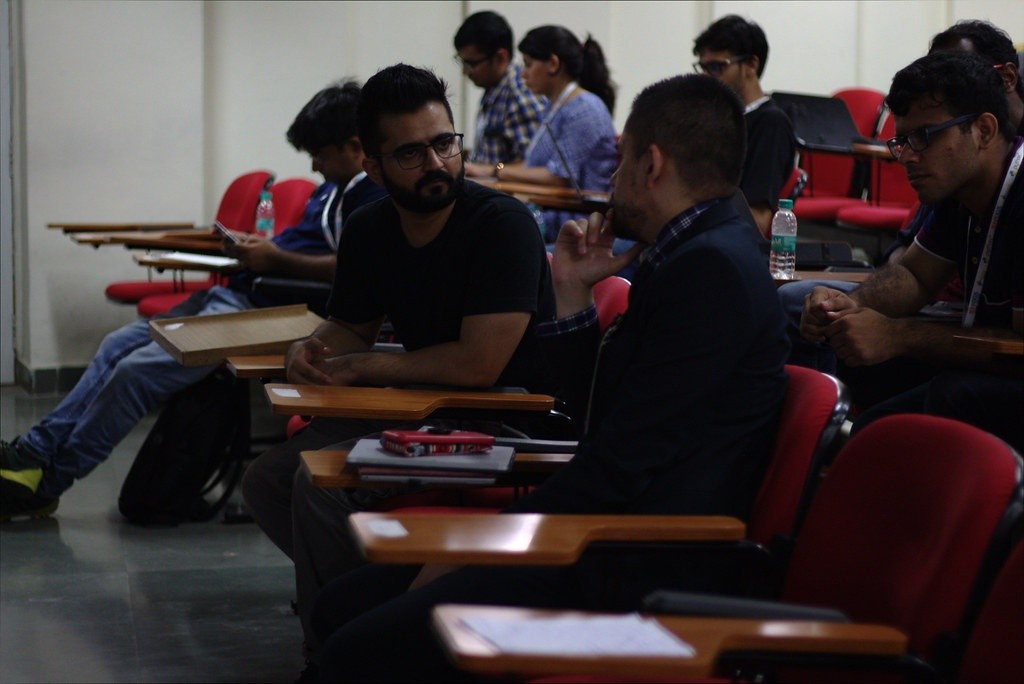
118, 365, 253, 524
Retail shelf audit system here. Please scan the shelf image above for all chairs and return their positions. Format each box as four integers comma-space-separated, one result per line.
49, 91, 1024, 684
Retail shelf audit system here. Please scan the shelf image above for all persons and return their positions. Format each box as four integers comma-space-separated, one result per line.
296, 72, 791, 684
454, 11, 548, 166
883, 20, 1024, 269
464, 26, 619, 244
241, 64, 557, 660
799, 51, 1024, 452
692, 15, 798, 234
1, 83, 384, 519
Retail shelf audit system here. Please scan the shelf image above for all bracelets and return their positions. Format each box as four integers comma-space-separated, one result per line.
493, 162, 504, 177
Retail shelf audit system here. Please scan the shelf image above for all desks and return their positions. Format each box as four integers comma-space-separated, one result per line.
299, 448, 576, 487
263, 381, 552, 422
474, 178, 572, 202
347, 511, 748, 563
225, 355, 287, 380
430, 603, 907, 677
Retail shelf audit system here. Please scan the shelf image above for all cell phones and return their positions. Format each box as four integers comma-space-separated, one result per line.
382, 430, 494, 446
213, 221, 240, 245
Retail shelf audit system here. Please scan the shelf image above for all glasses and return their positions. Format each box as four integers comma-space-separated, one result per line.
454, 51, 491, 70
886, 111, 981, 161
380, 132, 465, 170
693, 52, 751, 78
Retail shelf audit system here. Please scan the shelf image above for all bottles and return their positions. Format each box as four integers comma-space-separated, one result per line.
770, 199, 796, 280
255, 191, 274, 240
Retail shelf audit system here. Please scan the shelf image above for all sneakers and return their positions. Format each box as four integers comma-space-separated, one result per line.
0, 437, 67, 523
